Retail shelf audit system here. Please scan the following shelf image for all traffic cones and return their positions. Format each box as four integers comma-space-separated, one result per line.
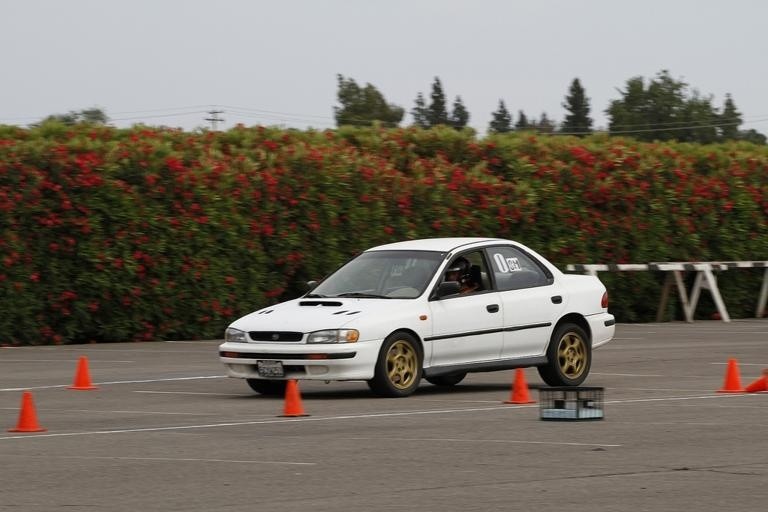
8, 391, 47, 433
503, 368, 536, 404
716, 359, 768, 393
276, 380, 310, 417
67, 356, 98, 389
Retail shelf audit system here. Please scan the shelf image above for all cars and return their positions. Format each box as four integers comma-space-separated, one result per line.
218, 237, 616, 397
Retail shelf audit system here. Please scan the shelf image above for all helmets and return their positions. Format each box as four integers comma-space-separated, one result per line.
445, 256, 471, 284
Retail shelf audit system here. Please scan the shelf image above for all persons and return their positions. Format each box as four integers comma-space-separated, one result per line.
443, 257, 477, 295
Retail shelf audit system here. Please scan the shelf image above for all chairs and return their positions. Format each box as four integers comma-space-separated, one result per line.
508, 270, 542, 289
